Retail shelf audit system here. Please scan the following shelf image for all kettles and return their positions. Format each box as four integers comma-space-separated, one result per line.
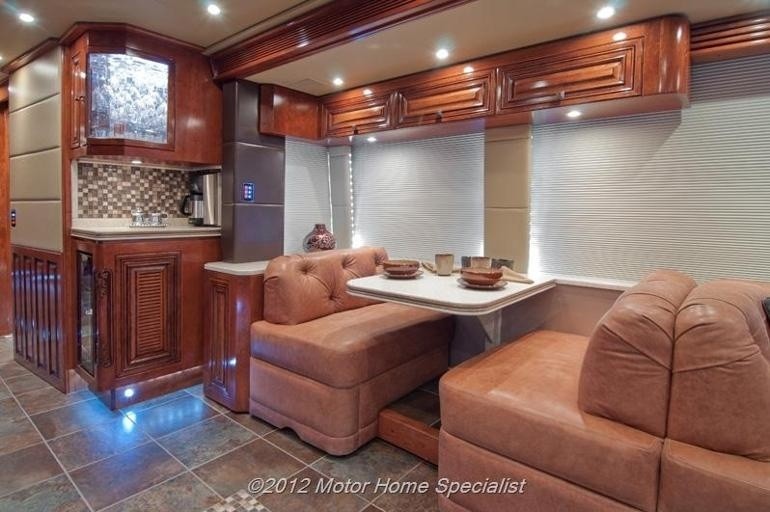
180, 190, 204, 226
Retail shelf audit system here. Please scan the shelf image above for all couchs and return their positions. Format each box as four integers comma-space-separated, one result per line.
437, 269, 698, 512
250, 246, 462, 456
657, 278, 770, 512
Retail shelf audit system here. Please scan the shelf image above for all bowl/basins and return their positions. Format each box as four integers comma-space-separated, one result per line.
460, 267, 503, 285
382, 259, 419, 275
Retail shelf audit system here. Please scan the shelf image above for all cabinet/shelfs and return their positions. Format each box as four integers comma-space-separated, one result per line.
73, 237, 233, 393
317, 13, 690, 147
202, 270, 264, 413
68, 29, 222, 165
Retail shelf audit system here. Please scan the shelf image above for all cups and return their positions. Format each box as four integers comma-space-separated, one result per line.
132, 208, 162, 225
471, 256, 492, 269
435, 254, 453, 276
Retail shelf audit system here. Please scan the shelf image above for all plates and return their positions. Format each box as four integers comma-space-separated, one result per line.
380, 270, 424, 279
456, 278, 507, 289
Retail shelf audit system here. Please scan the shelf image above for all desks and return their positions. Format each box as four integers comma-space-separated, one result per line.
346, 265, 557, 351
